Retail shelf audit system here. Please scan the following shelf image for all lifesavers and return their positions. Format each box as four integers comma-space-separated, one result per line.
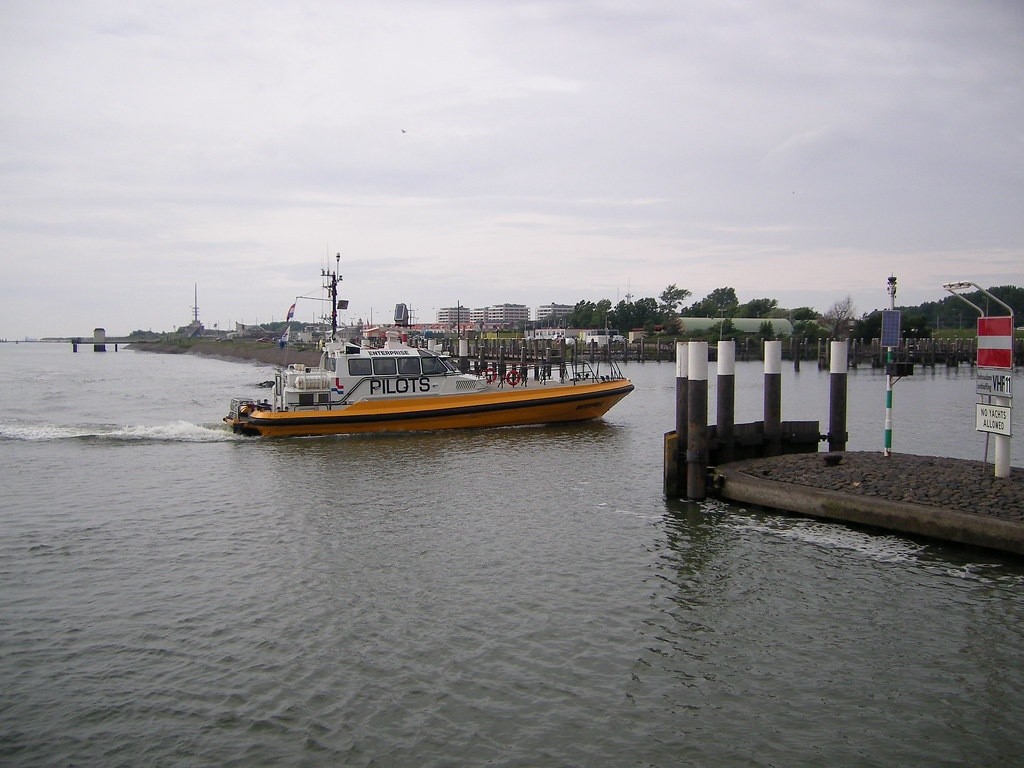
485, 368, 497, 382
506, 370, 520, 386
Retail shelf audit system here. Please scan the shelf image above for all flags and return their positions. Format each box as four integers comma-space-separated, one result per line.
278, 326, 290, 350
287, 304, 295, 322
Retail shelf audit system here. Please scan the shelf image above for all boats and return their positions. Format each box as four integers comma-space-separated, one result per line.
222, 252, 635, 436
